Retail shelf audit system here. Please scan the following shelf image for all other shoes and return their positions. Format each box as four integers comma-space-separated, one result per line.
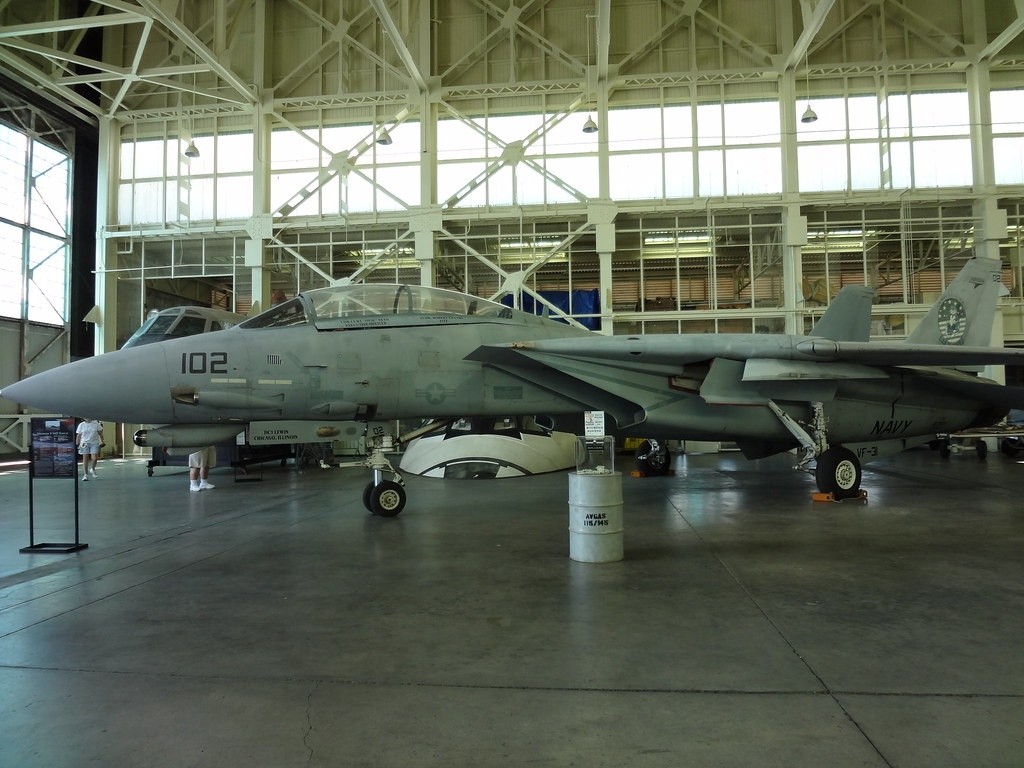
90, 468, 98, 478
82, 476, 88, 481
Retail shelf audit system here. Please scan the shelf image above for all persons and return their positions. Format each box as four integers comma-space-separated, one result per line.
76, 417, 106, 480
188, 445, 217, 492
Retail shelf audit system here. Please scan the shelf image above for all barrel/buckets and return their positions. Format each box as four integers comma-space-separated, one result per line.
568, 469, 624, 563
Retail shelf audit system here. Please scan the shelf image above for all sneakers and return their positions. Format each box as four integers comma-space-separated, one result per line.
190, 485, 201, 492
199, 483, 215, 489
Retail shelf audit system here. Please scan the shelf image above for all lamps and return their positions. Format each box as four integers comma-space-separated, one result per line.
376, 30, 392, 145
801, 50, 818, 122
184, 52, 201, 157
583, 15, 600, 133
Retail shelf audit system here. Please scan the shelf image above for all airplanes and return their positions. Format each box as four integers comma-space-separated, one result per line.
116, 299, 260, 351
0, 257, 1024, 519
928, 418, 1024, 459
